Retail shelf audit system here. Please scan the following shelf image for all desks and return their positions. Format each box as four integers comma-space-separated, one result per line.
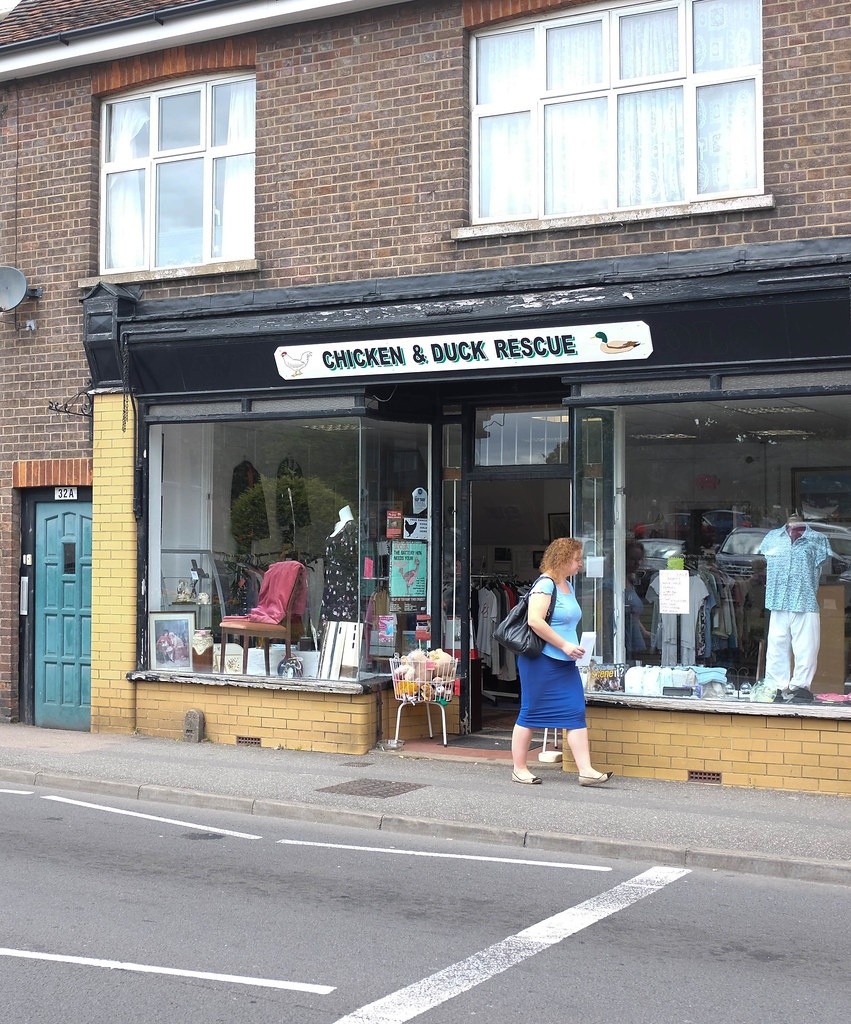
173, 603, 228, 630
371, 656, 461, 676
792, 580, 851, 694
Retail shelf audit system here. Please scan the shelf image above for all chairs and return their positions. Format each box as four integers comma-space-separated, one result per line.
219, 563, 303, 676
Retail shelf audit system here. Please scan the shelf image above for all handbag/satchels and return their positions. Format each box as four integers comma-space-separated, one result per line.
489, 576, 558, 660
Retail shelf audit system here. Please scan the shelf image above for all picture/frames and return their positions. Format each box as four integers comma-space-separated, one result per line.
791, 466, 851, 522
533, 551, 545, 568
148, 611, 198, 672
548, 512, 570, 544
393, 631, 430, 659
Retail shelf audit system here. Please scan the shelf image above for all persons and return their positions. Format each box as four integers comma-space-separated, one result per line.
156, 629, 185, 662
759, 514, 832, 690
509, 537, 614, 784
604, 541, 650, 663
318, 505, 358, 634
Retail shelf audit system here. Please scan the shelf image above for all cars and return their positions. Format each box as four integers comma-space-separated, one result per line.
787, 521, 851, 585
629, 538, 687, 597
570, 536, 611, 581
633, 510, 755, 534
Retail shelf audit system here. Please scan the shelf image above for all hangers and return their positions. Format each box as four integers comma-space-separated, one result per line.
665, 554, 725, 577
442, 572, 529, 591
211, 550, 324, 582
234, 455, 257, 473
279, 451, 299, 466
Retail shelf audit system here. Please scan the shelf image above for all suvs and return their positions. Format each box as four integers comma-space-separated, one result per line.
714, 526, 772, 581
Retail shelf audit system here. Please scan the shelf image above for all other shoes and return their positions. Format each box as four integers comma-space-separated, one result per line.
511, 770, 543, 785
577, 772, 615, 785
